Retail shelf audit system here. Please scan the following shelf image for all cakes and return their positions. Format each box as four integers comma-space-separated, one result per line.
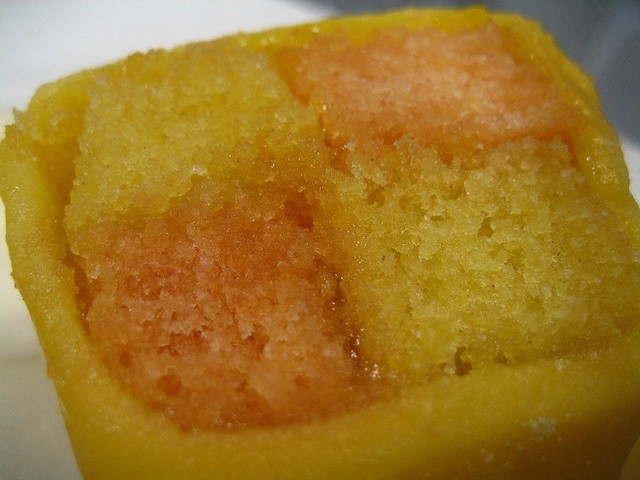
0, 5, 640, 480
